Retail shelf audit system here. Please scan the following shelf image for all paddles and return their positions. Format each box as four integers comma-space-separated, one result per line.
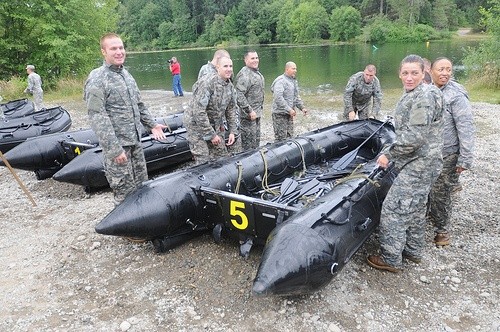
258, 171, 352, 205
332, 115, 393, 171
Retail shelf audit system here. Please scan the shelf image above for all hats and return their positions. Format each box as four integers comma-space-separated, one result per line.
26, 65, 35, 70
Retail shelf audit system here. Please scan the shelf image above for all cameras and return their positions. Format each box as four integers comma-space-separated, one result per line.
167, 59, 173, 64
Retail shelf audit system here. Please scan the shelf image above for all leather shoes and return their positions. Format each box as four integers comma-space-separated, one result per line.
367, 255, 401, 273
435, 233, 449, 246
402, 251, 422, 263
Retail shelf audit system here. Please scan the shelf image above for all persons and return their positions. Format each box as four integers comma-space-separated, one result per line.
83, 34, 168, 242
185, 56, 237, 166
198, 49, 241, 153
367, 53, 447, 273
169, 57, 183, 98
270, 61, 307, 141
234, 50, 265, 149
431, 56, 475, 246
343, 64, 381, 120
24, 65, 46, 112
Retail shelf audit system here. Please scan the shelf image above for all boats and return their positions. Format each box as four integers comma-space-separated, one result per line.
0, 98, 73, 152
95, 117, 401, 297
0, 112, 193, 191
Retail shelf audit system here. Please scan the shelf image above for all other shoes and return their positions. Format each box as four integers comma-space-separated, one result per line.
172, 96, 177, 98
126, 235, 147, 242
